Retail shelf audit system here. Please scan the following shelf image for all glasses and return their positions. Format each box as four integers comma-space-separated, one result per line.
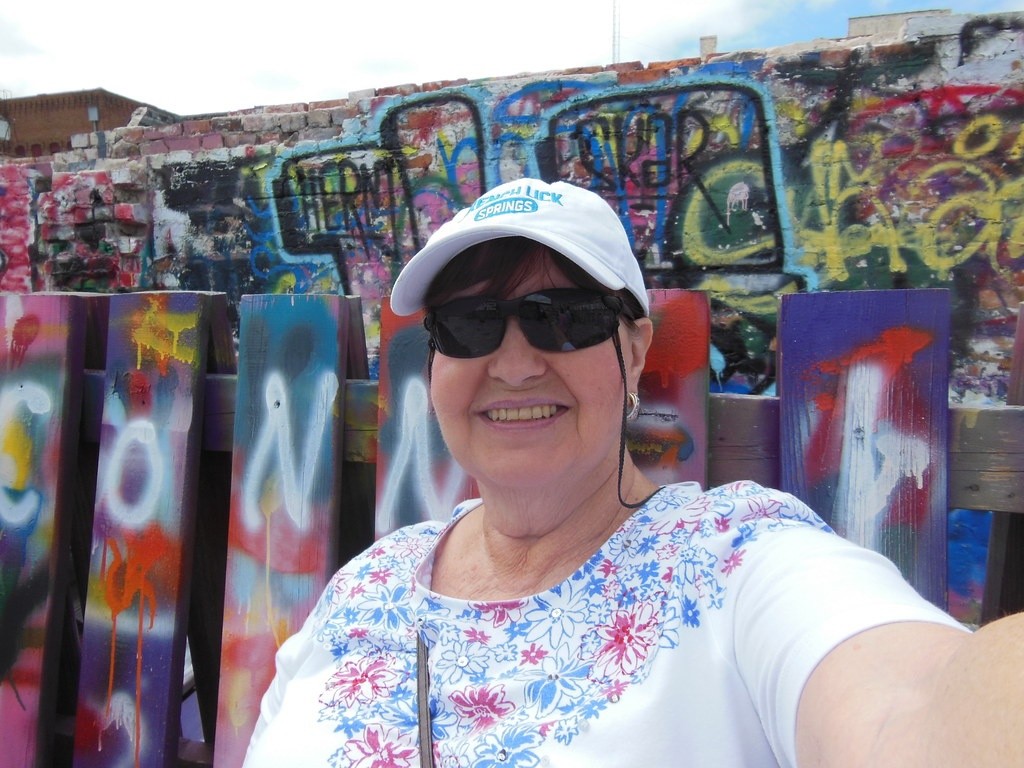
425, 287, 624, 360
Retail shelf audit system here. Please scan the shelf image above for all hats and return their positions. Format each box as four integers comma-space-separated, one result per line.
389, 178, 649, 317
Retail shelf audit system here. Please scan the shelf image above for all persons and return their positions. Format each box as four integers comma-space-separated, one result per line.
242, 177, 1022, 768
